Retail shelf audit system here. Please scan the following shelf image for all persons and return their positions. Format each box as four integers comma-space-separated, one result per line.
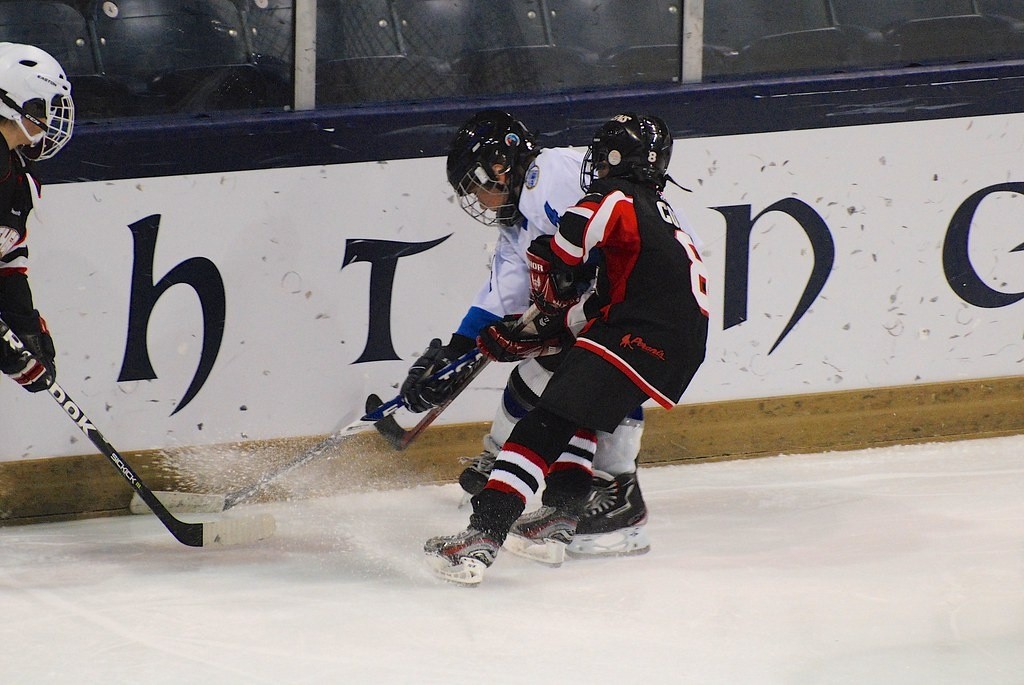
0, 42, 73, 392
420, 114, 708, 582
402, 109, 650, 556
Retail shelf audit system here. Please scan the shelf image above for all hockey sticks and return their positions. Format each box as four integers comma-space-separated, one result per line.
0, 317, 278, 548
364, 268, 575, 453
128, 345, 485, 515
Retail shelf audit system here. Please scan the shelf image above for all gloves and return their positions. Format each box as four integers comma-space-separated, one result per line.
477, 314, 564, 362
0, 308, 56, 394
405, 333, 483, 413
527, 234, 582, 310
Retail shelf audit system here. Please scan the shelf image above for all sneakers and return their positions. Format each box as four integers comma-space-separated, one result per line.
503, 502, 585, 564
567, 470, 651, 554
457, 435, 504, 508
424, 525, 497, 584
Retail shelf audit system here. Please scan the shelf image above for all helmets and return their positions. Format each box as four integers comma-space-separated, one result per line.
447, 109, 541, 228
0, 41, 75, 161
579, 113, 673, 193
